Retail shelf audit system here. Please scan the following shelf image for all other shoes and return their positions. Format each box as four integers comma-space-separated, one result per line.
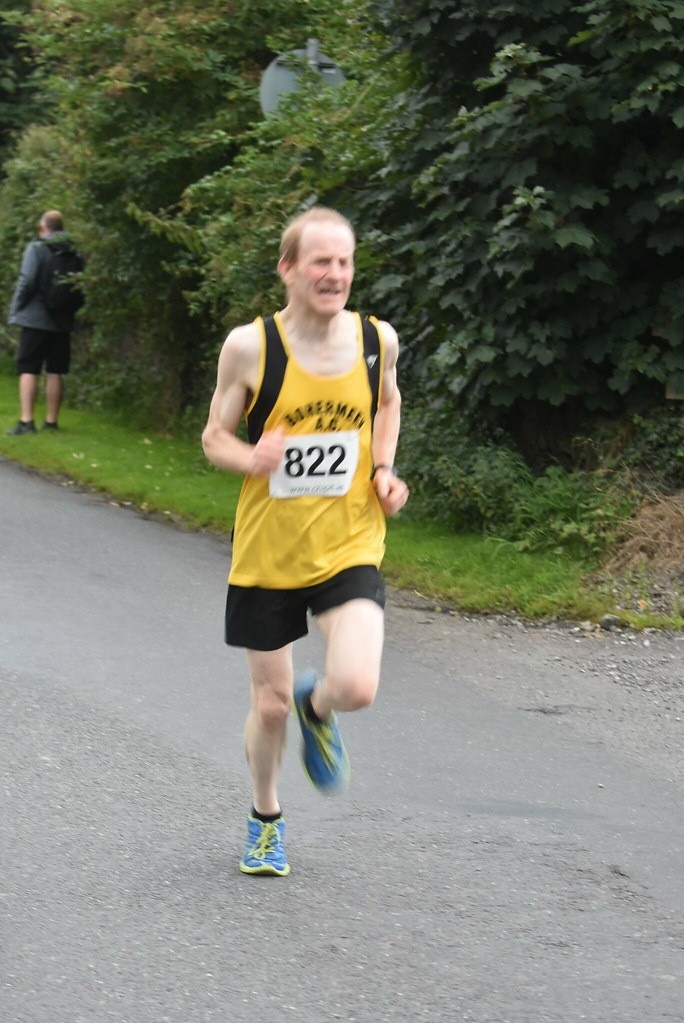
40, 422, 59, 433
8, 421, 37, 436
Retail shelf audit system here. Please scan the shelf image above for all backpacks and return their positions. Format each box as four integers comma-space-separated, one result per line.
35, 239, 86, 329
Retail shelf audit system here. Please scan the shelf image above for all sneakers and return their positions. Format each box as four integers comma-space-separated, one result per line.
290, 673, 349, 798
239, 812, 291, 877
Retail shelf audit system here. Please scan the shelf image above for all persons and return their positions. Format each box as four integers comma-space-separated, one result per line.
9, 209, 72, 434
199, 205, 406, 876
